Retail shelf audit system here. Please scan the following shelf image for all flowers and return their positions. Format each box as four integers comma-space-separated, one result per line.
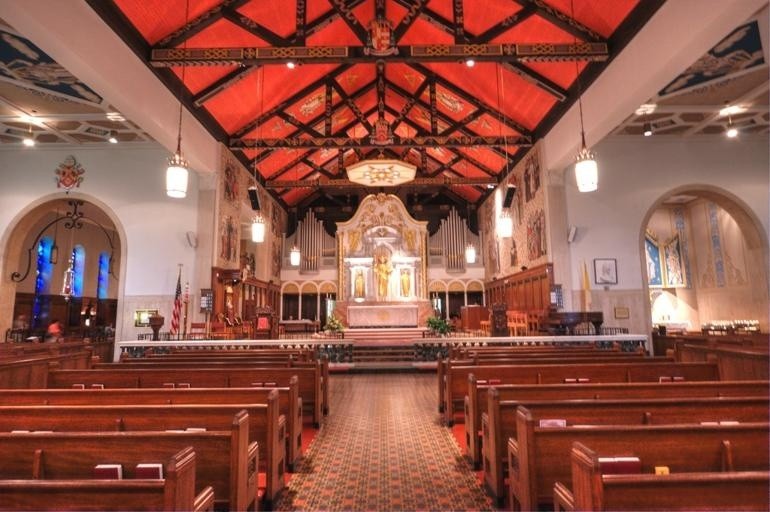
322, 312, 347, 334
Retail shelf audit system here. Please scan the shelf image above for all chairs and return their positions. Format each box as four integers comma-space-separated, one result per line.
506, 309, 544, 337
189, 320, 254, 338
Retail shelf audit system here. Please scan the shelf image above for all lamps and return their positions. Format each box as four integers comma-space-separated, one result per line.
345, 149, 418, 188
251, 65, 267, 242
289, 147, 301, 266
568, 1, 599, 195
164, 2, 191, 199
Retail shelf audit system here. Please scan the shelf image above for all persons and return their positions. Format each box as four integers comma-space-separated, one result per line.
48, 318, 66, 339
355, 262, 411, 299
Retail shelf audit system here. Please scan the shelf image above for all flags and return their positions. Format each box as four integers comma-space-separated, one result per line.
170, 278, 182, 335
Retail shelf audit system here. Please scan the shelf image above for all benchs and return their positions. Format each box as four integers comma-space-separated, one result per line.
1, 334, 331, 511
435, 324, 769, 511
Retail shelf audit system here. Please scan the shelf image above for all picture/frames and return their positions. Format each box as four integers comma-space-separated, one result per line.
594, 258, 618, 285
